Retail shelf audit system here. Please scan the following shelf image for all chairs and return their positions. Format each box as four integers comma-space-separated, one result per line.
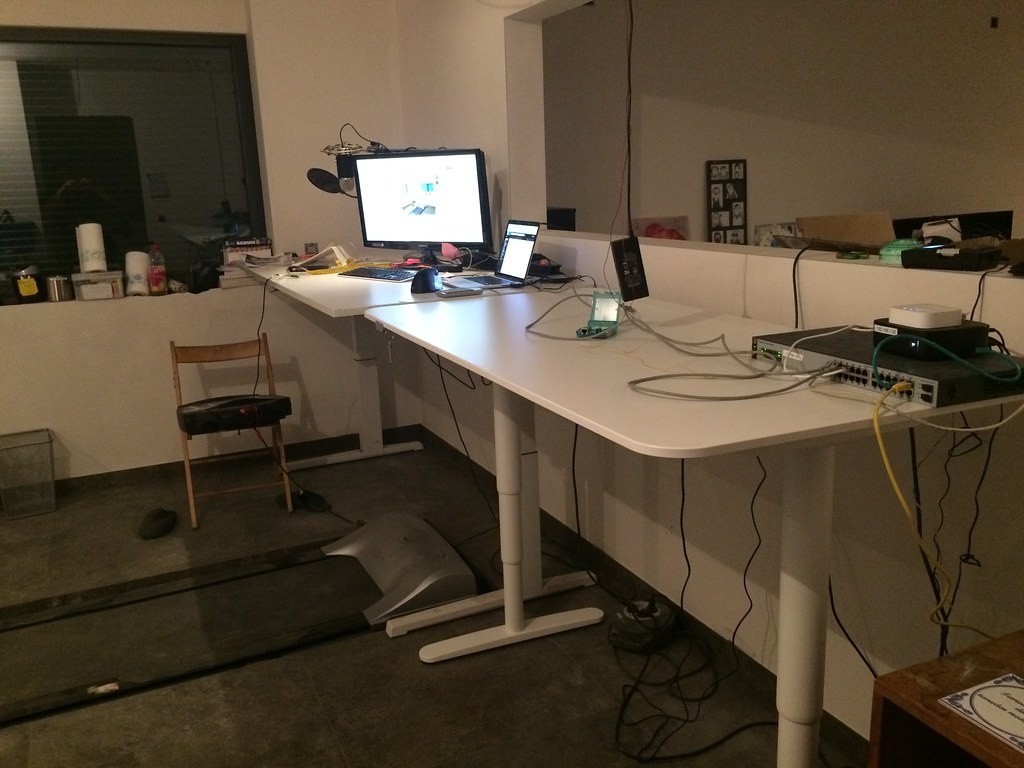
170, 332, 292, 530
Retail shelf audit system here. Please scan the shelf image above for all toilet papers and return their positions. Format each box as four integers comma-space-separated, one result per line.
125, 251, 151, 296
75, 222, 107, 273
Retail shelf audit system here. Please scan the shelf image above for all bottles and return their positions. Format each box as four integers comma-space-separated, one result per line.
147, 244, 167, 295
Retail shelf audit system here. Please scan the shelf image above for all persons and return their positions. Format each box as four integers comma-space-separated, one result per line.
711, 165, 743, 245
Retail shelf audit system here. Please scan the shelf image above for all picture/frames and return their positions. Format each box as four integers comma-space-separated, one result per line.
705, 159, 747, 245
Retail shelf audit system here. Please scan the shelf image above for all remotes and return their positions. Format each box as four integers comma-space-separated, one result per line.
435, 288, 483, 298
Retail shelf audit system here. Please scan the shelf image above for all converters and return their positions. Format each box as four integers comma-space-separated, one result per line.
628, 593, 656, 617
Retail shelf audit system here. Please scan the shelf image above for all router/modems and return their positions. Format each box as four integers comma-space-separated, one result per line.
872, 317, 990, 360
889, 302, 963, 329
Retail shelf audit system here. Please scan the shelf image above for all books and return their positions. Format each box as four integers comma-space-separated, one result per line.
214, 237, 272, 288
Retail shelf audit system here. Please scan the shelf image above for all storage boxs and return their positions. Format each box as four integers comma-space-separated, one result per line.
72, 271, 124, 301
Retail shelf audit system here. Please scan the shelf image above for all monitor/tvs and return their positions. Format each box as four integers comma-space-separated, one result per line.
349, 150, 493, 271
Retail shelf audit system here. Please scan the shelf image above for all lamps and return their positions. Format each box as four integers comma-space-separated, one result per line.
321, 122, 386, 192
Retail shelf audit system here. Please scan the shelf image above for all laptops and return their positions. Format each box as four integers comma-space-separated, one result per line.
442, 220, 541, 290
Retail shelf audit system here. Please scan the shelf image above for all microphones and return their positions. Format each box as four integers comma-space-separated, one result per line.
335, 154, 355, 192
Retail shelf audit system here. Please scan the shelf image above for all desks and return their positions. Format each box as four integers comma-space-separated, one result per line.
232, 250, 1024, 768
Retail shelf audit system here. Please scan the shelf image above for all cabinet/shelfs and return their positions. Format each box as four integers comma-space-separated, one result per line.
867, 628, 1024, 768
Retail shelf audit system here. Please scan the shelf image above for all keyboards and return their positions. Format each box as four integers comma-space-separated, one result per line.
338, 266, 416, 283
